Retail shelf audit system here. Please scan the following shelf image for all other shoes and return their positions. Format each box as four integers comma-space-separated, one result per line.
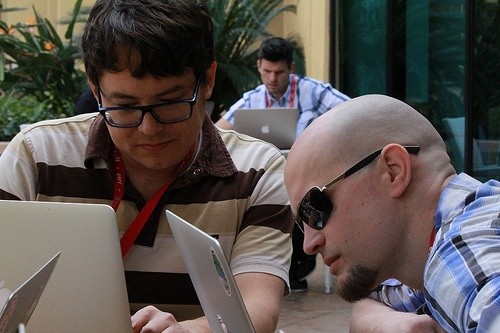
290, 280, 307, 292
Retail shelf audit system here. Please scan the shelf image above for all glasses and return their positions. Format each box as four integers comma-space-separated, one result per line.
94, 72, 202, 127
293, 146, 420, 234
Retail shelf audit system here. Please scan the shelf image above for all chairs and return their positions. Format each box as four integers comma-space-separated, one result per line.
442, 116, 500, 184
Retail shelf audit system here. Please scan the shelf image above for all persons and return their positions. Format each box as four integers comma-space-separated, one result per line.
283, 94, 500, 333
0, 0, 295, 333
213, 37, 352, 292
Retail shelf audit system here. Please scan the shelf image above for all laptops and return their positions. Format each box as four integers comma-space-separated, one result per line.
165, 210, 300, 333
233, 107, 300, 149
0, 199, 134, 333
0, 249, 60, 333
